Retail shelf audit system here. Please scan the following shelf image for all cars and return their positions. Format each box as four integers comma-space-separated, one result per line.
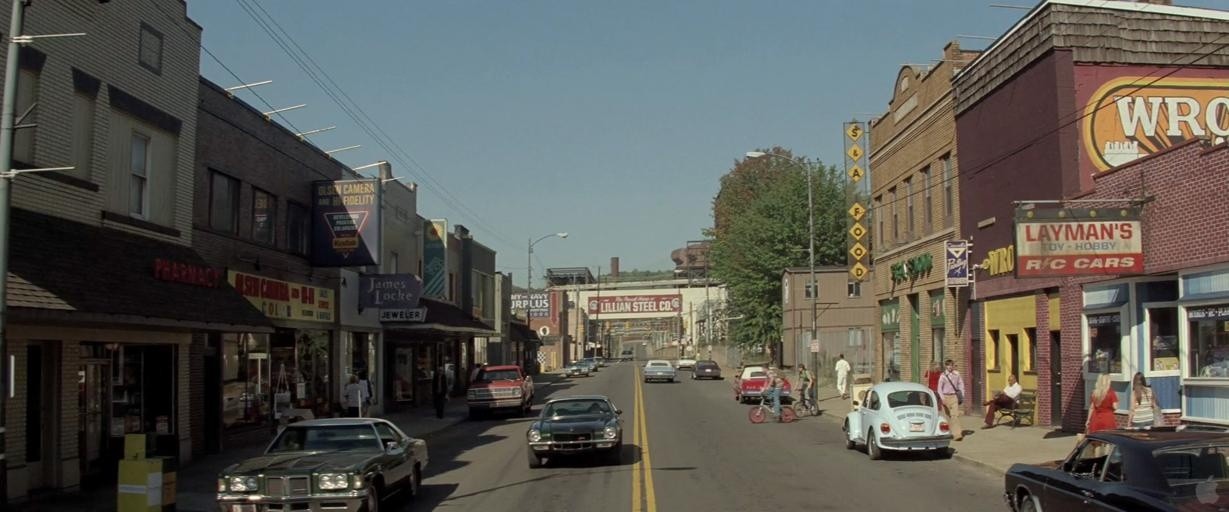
1001, 424, 1229, 510
691, 359, 722, 381
214, 417, 430, 512
620, 350, 634, 360
841, 378, 956, 461
593, 356, 606, 368
525, 394, 625, 471
563, 359, 590, 377
640, 359, 677, 383
730, 360, 792, 404
580, 357, 599, 372
675, 355, 696, 370
465, 363, 535, 417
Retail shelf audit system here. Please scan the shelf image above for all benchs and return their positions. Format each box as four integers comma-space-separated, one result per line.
993, 389, 1039, 427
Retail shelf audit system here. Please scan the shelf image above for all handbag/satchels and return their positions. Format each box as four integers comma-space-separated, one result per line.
957, 390, 963, 404
1075, 428, 1096, 459
1153, 406, 1164, 427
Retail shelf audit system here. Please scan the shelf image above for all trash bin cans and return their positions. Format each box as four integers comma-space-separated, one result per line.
850, 374, 874, 410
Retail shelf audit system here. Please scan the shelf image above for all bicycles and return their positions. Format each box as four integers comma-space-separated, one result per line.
746, 394, 794, 425
793, 388, 822, 418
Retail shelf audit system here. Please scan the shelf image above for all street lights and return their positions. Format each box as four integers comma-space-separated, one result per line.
527, 229, 569, 326
672, 268, 713, 346
741, 151, 819, 401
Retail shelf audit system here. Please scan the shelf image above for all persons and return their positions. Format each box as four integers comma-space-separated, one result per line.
1127, 371, 1157, 429
793, 363, 814, 409
938, 360, 965, 440
1084, 371, 1119, 433
344, 375, 362, 417
1204, 351, 1228, 377
834, 354, 851, 399
431, 367, 449, 419
469, 363, 482, 383
762, 367, 784, 421
980, 374, 1023, 428
924, 359, 942, 411
